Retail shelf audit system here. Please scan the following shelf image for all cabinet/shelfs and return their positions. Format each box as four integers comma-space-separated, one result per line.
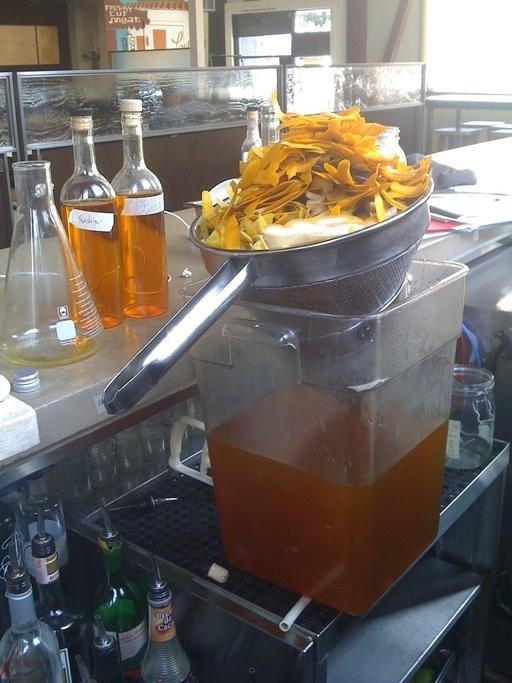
62, 430, 511, 683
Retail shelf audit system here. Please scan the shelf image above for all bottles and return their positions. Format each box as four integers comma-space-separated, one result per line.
27, 506, 83, 681
88, 525, 149, 668
108, 96, 172, 321
236, 106, 281, 165
57, 106, 128, 331
1, 468, 72, 587
1, 565, 66, 683
0, 159, 109, 369
136, 582, 194, 682
82, 616, 126, 683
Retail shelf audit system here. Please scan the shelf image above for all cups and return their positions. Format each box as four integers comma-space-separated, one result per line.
442, 364, 497, 476
